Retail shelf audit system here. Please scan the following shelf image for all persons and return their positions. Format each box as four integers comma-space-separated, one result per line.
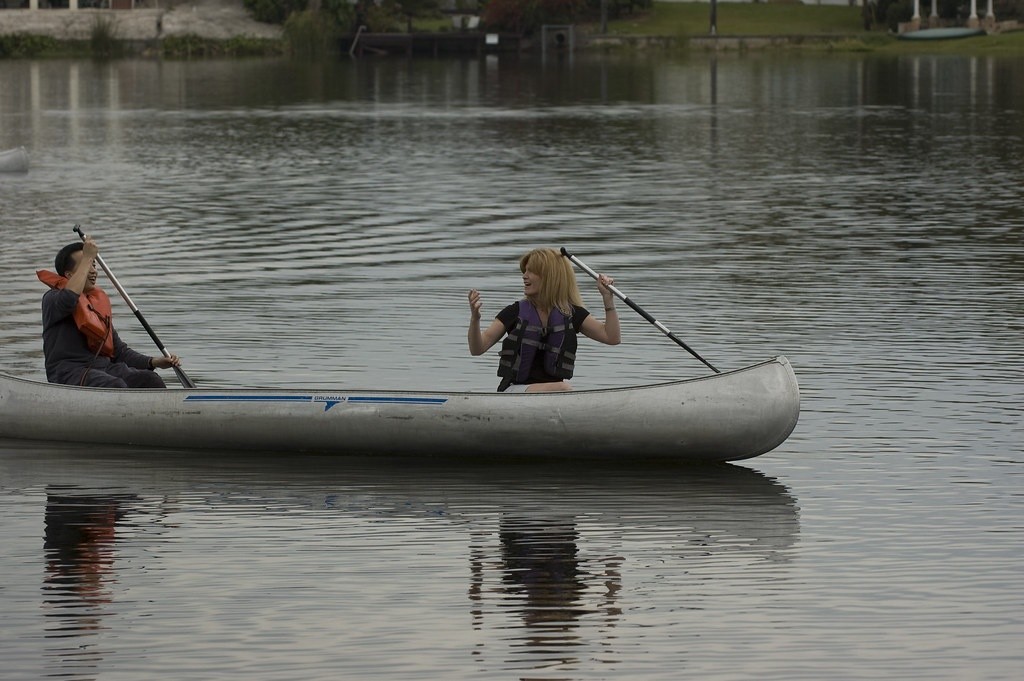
42, 234, 181, 388
468, 248, 621, 392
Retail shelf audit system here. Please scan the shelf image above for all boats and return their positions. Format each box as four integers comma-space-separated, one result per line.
0, 146, 29, 173
0, 352, 803, 465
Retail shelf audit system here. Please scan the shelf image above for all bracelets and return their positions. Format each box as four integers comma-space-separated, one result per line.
605, 306, 617, 310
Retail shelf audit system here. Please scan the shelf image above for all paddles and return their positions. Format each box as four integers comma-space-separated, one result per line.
71, 220, 198, 389
559, 244, 723, 374
